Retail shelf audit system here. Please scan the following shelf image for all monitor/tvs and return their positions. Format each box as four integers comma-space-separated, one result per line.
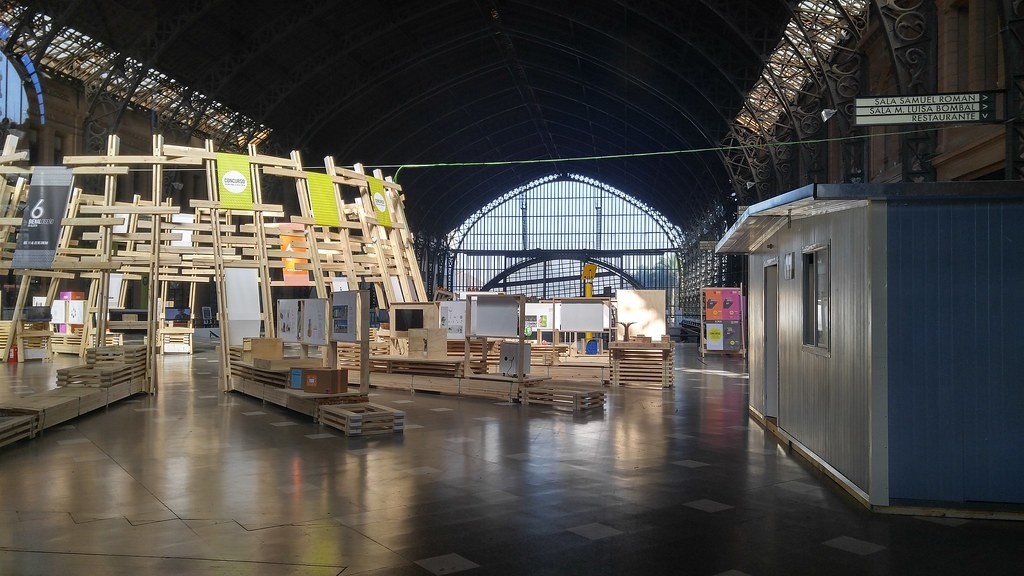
165, 307, 191, 321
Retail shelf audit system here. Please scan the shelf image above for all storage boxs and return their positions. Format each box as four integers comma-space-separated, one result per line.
631, 335, 652, 343
289, 366, 331, 389
251, 338, 322, 371
122, 314, 138, 322
60, 291, 84, 300
303, 369, 348, 394
241, 348, 252, 364
243, 336, 262, 351
661, 335, 670, 342
581, 337, 604, 355
408, 328, 447, 359
